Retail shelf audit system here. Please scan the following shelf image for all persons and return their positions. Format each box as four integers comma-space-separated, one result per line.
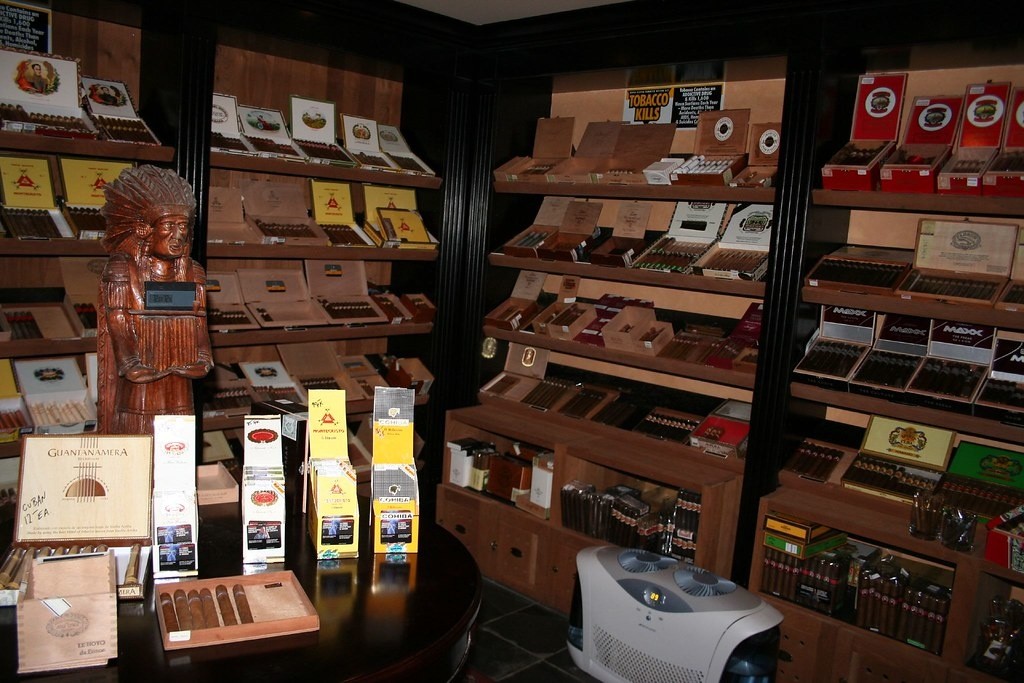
97, 165, 215, 437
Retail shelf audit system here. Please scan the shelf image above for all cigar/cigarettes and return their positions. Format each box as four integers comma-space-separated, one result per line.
0, 101, 1024, 683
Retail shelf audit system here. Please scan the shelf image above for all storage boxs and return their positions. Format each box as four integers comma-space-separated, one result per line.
482, 69, 1024, 543
2, 45, 441, 668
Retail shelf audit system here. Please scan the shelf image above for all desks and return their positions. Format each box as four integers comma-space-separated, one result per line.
0, 497, 483, 683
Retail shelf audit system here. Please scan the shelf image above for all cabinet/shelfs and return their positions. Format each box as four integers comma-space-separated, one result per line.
790, 167, 1024, 446
0, 128, 178, 459
544, 527, 595, 618
761, 596, 951, 683
435, 483, 551, 613
208, 137, 442, 498
475, 163, 776, 404
948, 667, 999, 683
